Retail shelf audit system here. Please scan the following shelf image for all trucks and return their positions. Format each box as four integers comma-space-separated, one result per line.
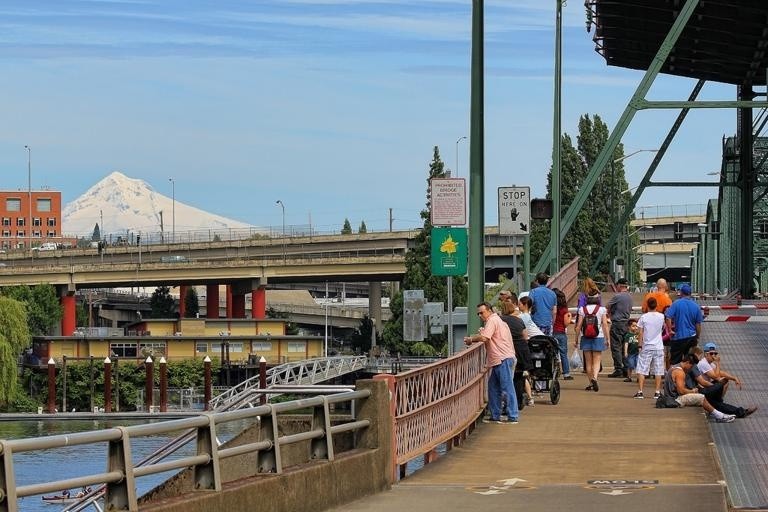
159, 255, 191, 263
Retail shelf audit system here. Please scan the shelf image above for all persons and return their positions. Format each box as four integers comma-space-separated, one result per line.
605, 278, 633, 379
464, 301, 518, 425
624, 278, 760, 423
488, 271, 574, 420
136, 235, 139, 244
573, 286, 611, 392
574, 277, 603, 332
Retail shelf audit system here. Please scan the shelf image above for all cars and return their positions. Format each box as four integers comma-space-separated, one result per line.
33, 239, 128, 251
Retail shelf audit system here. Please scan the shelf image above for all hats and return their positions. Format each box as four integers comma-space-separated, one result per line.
704, 343, 716, 351
588, 293, 599, 298
678, 283, 691, 294
615, 278, 629, 285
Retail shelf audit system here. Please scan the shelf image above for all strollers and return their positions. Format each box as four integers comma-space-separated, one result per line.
523, 334, 561, 405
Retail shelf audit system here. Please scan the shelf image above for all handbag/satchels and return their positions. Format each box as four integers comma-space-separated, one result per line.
564, 312, 572, 327
656, 394, 681, 408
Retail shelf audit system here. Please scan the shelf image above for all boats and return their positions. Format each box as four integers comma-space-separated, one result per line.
41, 495, 82, 505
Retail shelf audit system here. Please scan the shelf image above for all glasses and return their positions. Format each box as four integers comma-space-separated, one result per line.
705, 352, 718, 356
477, 310, 488, 315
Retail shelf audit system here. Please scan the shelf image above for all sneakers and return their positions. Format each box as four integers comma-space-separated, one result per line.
654, 392, 661, 398
608, 370, 632, 382
586, 377, 599, 391
482, 397, 534, 424
744, 406, 757, 418
709, 413, 736, 423
564, 376, 573, 380
632, 392, 644, 399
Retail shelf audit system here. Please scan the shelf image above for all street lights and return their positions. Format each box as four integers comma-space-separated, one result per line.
274, 200, 286, 259
168, 177, 177, 243
455, 135, 468, 176
608, 146, 657, 288
24, 145, 32, 251
679, 168, 727, 296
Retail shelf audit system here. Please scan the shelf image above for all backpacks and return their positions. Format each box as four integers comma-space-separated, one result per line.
583, 305, 600, 338
